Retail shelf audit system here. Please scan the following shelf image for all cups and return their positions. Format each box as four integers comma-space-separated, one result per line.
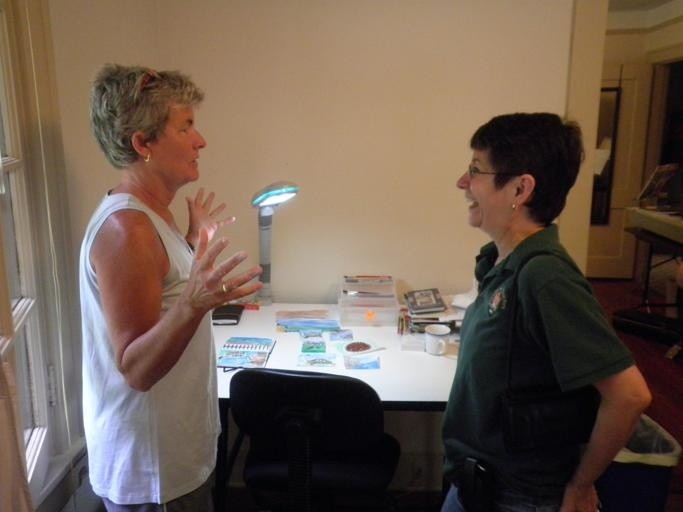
424, 323, 452, 356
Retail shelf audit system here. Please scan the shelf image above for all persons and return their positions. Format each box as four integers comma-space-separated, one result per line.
435, 112, 653, 511
78, 64, 265, 512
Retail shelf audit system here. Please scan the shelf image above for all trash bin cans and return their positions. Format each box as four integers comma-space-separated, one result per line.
595, 410, 682, 512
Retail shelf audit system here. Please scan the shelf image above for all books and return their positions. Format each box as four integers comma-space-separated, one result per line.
339, 275, 456, 332
215, 335, 276, 368
211, 305, 245, 326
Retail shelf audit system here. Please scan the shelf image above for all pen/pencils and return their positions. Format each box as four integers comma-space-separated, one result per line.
231, 305, 261, 310
401, 317, 404, 335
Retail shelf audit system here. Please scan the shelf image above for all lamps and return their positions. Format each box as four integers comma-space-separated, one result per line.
250, 179, 301, 306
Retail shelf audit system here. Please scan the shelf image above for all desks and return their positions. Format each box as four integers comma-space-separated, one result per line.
212, 301, 462, 511
620, 205, 682, 308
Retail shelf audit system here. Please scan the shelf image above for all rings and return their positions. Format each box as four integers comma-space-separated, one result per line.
222, 282, 227, 293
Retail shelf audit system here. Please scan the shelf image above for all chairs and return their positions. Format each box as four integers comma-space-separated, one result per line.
231, 369, 402, 512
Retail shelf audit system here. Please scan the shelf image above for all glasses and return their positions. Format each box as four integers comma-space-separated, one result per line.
469, 164, 495, 180
